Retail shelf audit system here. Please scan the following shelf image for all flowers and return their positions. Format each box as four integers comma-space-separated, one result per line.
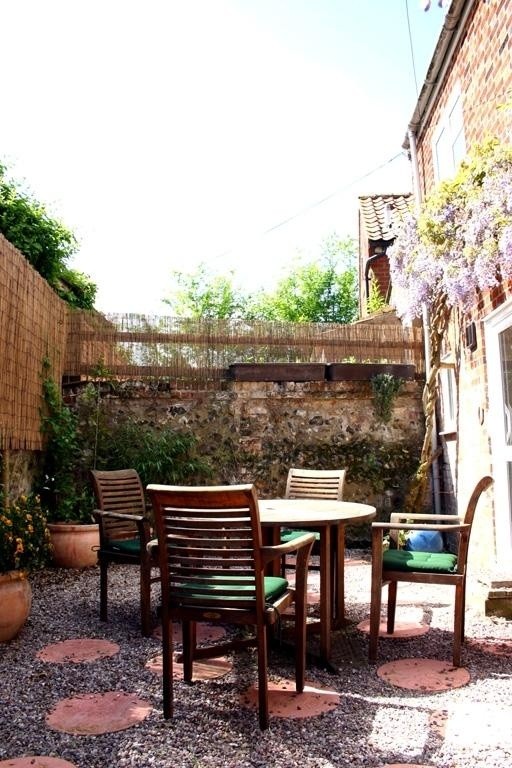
0, 493, 55, 582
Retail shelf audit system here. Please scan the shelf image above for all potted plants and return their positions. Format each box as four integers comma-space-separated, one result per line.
35, 372, 115, 570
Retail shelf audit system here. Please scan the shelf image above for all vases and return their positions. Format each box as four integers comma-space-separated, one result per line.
0, 569, 33, 643
